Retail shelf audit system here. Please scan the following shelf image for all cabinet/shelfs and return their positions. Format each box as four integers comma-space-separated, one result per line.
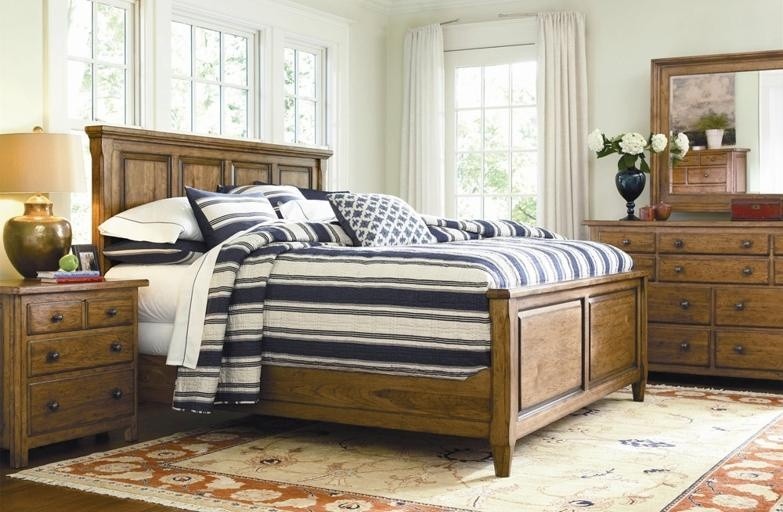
580, 218, 783, 384
672, 149, 751, 193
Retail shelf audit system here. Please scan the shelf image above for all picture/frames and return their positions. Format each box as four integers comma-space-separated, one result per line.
72, 244, 100, 275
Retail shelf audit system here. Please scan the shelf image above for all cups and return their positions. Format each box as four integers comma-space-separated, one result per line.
638, 199, 672, 221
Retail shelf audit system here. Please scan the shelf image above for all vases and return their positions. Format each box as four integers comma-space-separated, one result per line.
616, 163, 646, 221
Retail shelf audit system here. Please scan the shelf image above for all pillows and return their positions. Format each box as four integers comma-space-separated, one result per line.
278, 199, 336, 222
98, 197, 204, 244
101, 239, 204, 263
252, 179, 350, 200
324, 192, 437, 247
216, 182, 307, 219
183, 184, 279, 250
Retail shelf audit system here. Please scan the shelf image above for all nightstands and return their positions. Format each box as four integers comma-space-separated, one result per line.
0, 279, 150, 469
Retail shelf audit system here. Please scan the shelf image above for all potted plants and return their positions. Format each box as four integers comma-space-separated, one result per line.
697, 108, 728, 148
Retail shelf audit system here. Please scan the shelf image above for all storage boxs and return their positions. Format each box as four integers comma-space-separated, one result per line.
732, 198, 783, 220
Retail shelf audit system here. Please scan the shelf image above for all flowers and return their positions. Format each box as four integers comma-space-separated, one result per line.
670, 132, 690, 159
588, 129, 668, 173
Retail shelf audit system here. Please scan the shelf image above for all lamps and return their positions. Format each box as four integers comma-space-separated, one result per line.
1, 125, 85, 280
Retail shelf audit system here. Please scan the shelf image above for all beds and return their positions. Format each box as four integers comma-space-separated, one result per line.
86, 124, 647, 478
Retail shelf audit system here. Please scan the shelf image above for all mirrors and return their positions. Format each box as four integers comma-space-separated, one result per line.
648, 48, 783, 214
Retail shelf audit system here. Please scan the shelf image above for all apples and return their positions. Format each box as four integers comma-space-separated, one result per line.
58, 255, 79, 272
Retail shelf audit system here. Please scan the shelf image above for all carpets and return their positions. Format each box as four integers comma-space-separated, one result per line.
6, 382, 783, 512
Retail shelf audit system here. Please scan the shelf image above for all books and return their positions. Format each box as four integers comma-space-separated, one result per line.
37, 270, 106, 284
